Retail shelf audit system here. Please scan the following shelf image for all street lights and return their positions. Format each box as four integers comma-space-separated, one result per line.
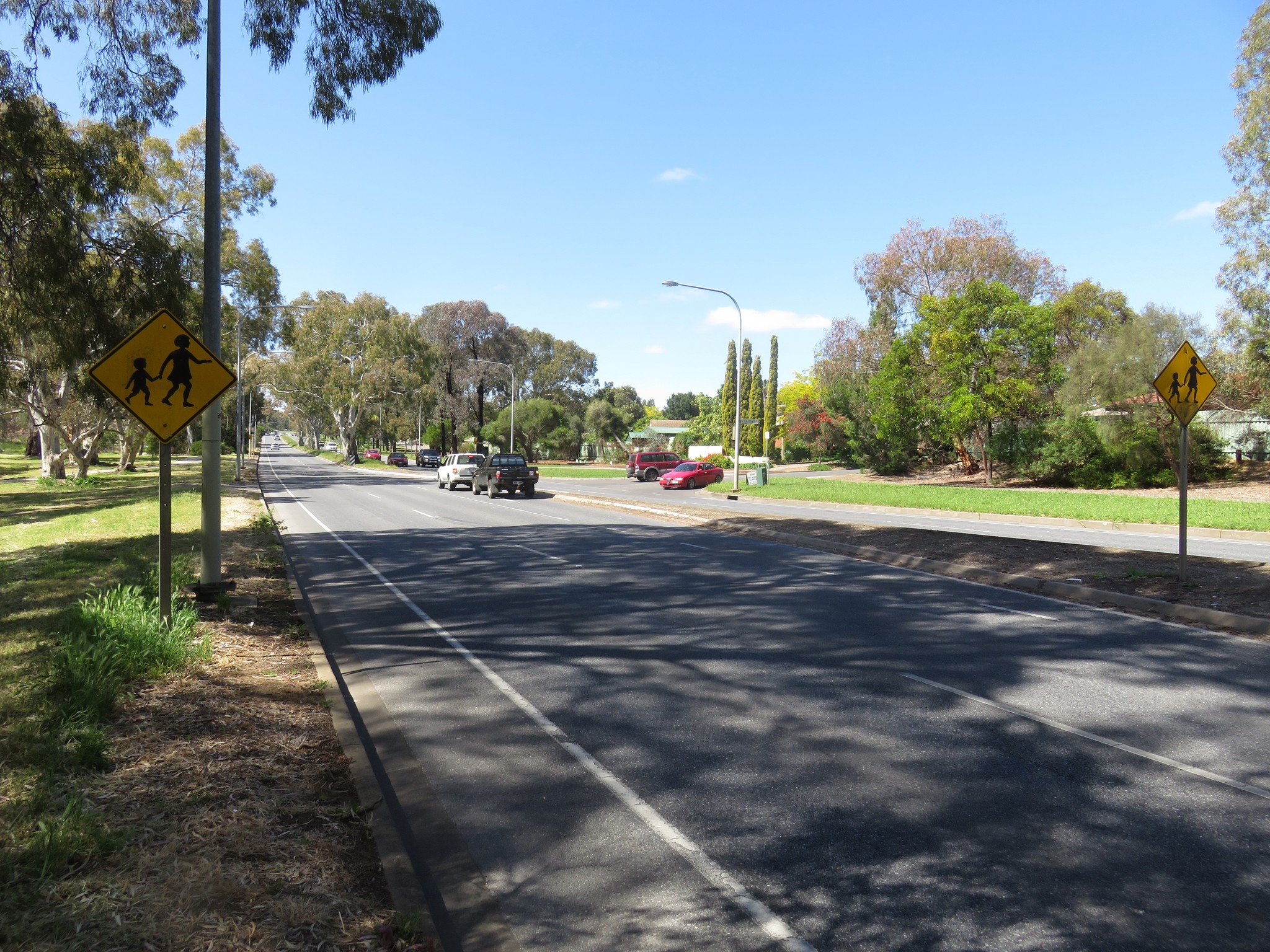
662, 280, 741, 489
236, 305, 317, 482
241, 352, 297, 469
248, 384, 286, 458
254, 406, 270, 450
468, 358, 514, 453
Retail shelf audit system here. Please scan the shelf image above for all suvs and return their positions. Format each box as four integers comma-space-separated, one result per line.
626, 453, 695, 483
437, 453, 486, 491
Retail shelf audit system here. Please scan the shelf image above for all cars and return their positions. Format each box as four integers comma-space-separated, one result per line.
325, 442, 338, 452
415, 450, 440, 468
265, 430, 285, 440
270, 443, 279, 450
364, 449, 382, 460
388, 452, 408, 467
659, 462, 723, 490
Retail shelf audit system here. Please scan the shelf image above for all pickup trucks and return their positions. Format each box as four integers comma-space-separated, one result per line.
471, 453, 538, 499
